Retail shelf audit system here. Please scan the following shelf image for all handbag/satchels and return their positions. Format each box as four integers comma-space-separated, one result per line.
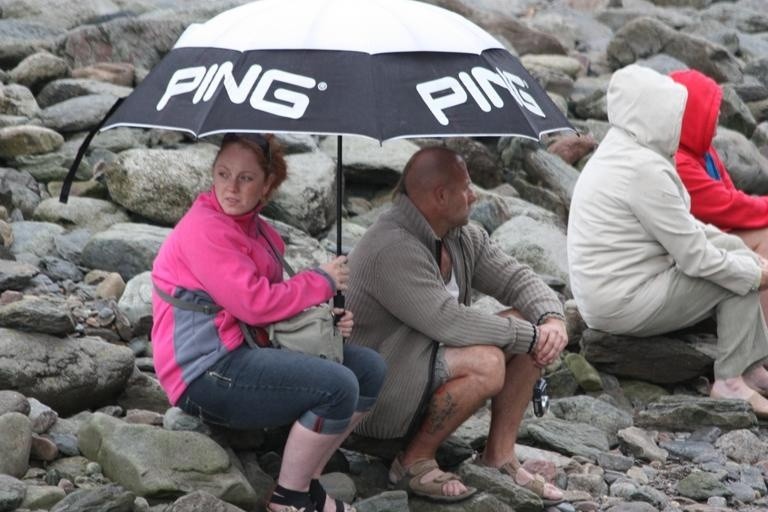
266, 298, 344, 364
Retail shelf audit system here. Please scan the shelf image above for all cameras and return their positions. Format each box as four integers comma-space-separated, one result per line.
533, 378, 550, 416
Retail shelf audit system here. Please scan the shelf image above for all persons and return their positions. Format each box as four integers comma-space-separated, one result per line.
341, 149, 570, 505
567, 64, 768, 417
148, 131, 387, 512
661, 71, 768, 321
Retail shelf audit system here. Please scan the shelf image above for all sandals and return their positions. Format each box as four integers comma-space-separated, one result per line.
267, 477, 356, 512
387, 451, 478, 503
474, 453, 565, 505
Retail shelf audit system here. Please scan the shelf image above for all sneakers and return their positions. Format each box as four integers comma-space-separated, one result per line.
711, 386, 768, 414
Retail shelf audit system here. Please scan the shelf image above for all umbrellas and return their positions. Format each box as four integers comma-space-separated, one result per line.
57, 1, 581, 330
